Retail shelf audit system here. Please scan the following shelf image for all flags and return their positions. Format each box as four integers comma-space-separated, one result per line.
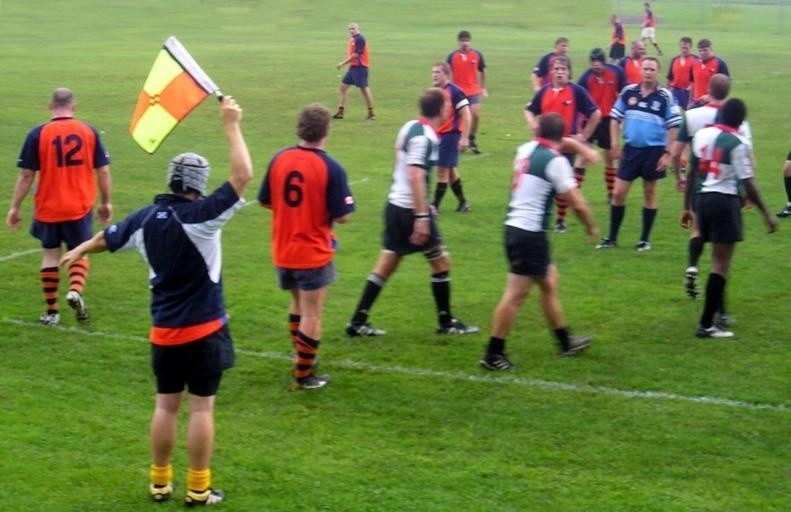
129, 43, 210, 156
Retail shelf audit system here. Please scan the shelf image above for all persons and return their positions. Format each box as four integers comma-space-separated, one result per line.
58, 94, 253, 508
256, 103, 356, 390
478, 4, 791, 370
7, 87, 113, 325
343, 30, 490, 338
335, 25, 376, 122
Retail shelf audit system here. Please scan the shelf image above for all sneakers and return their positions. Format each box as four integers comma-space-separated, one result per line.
333, 112, 343, 118
65, 291, 90, 322
150, 481, 175, 500
346, 321, 387, 336
293, 373, 329, 390
39, 314, 60, 327
469, 140, 480, 153
185, 487, 225, 507
776, 206, 791, 217
436, 319, 479, 335
368, 114, 375, 119
596, 240, 651, 252
429, 202, 469, 214
480, 355, 516, 371
685, 266, 701, 300
561, 336, 592, 355
694, 313, 734, 338
555, 221, 566, 233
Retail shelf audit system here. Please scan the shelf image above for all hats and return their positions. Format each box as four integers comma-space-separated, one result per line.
167, 152, 209, 197
